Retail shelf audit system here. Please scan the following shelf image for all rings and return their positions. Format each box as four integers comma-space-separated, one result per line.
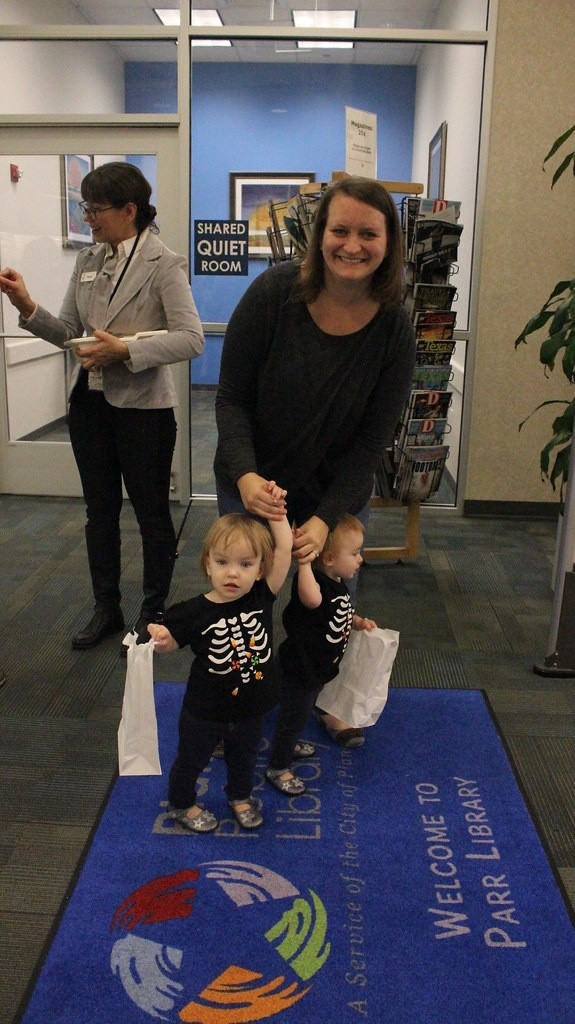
313, 551, 320, 558
92, 367, 97, 372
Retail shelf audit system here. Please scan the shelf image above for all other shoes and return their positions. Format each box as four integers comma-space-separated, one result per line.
213, 740, 225, 758
313, 708, 365, 747
293, 743, 315, 759
227, 798, 263, 830
264, 767, 305, 796
166, 803, 218, 833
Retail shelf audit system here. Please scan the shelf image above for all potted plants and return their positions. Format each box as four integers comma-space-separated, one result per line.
513, 125, 575, 592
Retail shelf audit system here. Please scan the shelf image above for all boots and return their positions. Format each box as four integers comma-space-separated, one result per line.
120, 541, 179, 657
71, 540, 125, 649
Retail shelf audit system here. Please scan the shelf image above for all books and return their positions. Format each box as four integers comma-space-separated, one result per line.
282, 177, 464, 502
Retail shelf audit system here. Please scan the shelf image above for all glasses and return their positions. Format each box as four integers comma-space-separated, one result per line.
78, 199, 116, 219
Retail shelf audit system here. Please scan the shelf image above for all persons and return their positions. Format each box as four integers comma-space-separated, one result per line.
0, 162, 205, 656
214, 179, 417, 747
267, 512, 376, 795
147, 481, 293, 831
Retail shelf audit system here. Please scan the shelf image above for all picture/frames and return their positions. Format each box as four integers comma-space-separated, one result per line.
229, 172, 317, 260
59, 154, 94, 251
425, 121, 447, 201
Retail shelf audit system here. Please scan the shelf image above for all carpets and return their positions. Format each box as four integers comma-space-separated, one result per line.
12, 679, 575, 1024
